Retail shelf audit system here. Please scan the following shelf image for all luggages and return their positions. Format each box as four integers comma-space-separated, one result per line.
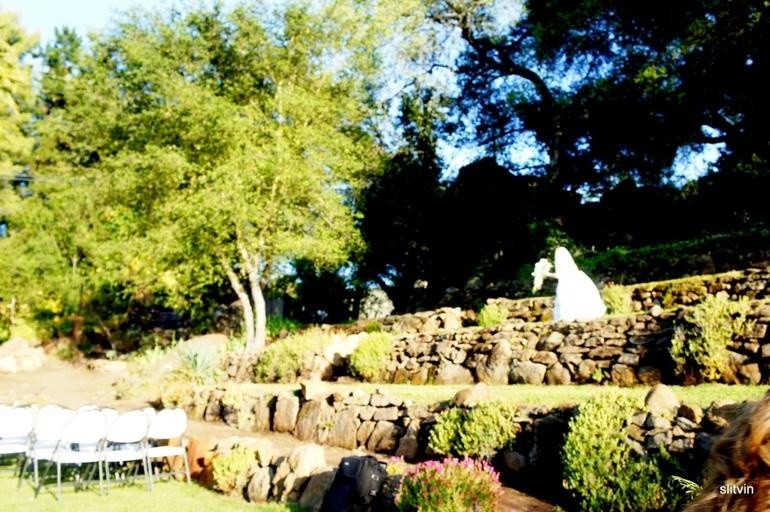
318, 455, 387, 512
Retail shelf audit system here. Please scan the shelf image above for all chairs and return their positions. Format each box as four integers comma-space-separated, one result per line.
0, 404, 191, 500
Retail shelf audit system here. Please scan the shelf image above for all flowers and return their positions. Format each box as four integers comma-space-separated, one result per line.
393, 456, 504, 512
